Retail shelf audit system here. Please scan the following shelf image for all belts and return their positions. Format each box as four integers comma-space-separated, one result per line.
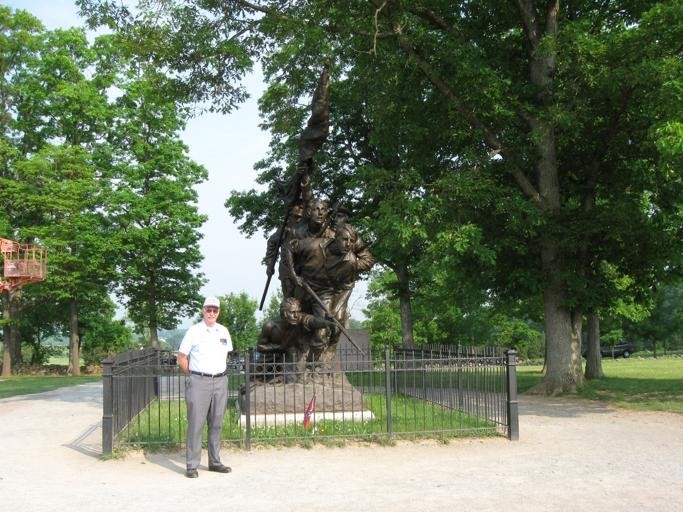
189, 370, 226, 378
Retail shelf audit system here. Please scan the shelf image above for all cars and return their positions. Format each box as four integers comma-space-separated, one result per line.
582, 339, 633, 359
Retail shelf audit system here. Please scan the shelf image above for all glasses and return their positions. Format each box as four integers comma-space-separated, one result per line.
204, 307, 219, 314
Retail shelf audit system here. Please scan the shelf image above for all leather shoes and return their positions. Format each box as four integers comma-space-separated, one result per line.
209, 465, 231, 473
187, 468, 198, 478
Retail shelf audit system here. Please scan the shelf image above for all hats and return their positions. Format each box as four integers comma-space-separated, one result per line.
203, 297, 220, 307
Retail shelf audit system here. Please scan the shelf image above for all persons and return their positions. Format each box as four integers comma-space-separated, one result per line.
176, 296, 233, 477
252, 295, 338, 382
264, 163, 373, 365
